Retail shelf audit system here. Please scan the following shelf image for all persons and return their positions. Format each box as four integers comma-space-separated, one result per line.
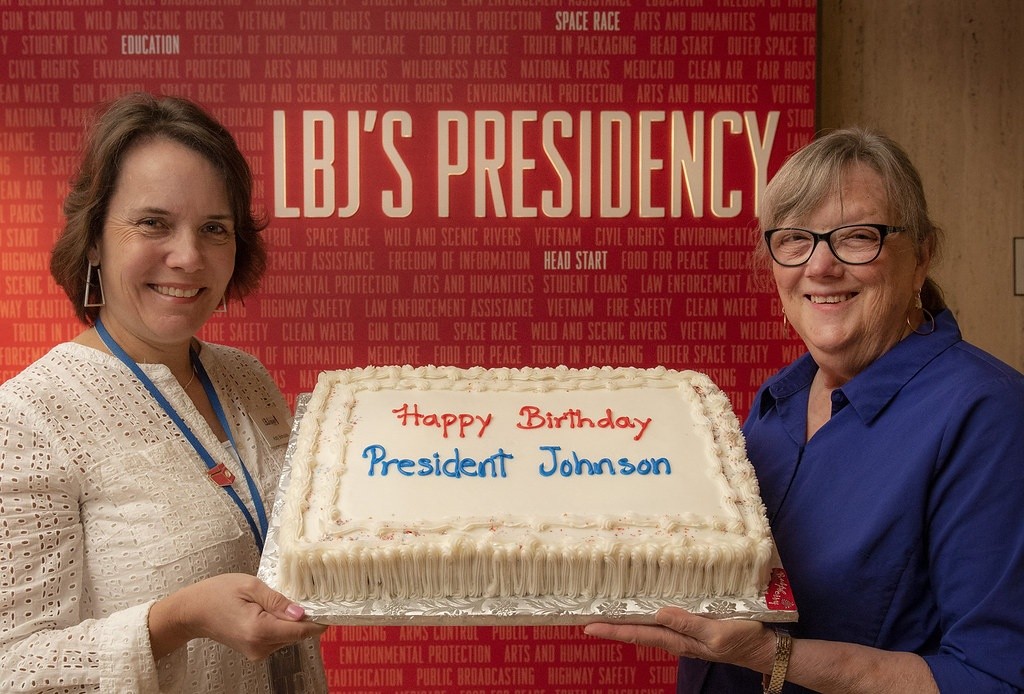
584, 128, 1024, 694
0, 92, 330, 694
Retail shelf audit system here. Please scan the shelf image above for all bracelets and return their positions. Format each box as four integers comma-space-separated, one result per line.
761, 630, 792, 694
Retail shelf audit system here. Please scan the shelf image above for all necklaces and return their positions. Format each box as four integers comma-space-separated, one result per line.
183, 368, 195, 389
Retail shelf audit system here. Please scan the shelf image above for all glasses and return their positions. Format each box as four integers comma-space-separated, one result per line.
764, 223, 910, 266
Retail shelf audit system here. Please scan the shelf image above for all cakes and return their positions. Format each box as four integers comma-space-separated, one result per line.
276, 365, 774, 597
